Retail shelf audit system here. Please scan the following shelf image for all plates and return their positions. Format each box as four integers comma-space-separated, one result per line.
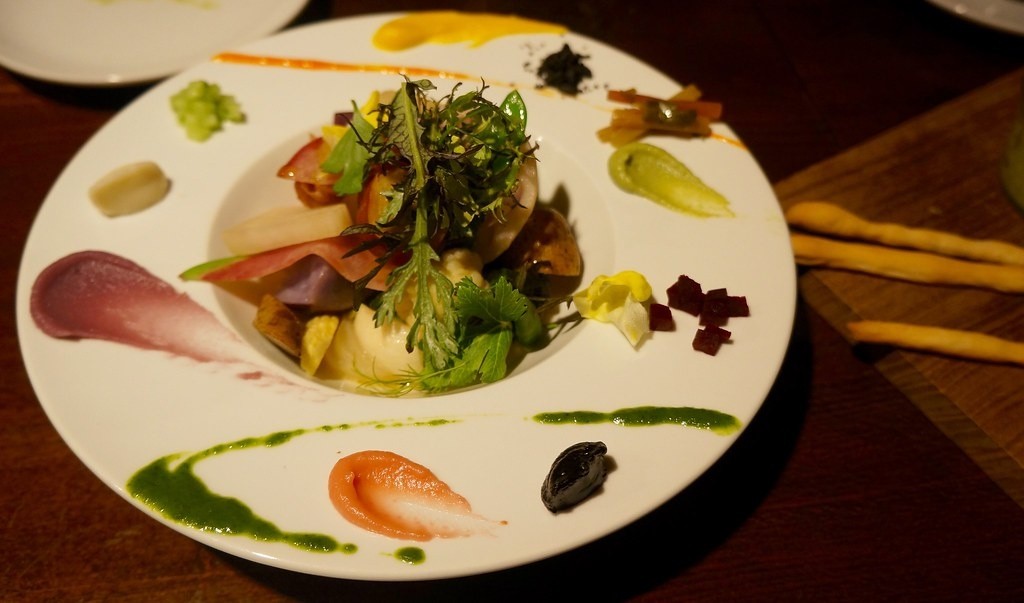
931, 0, 1024, 33
14, 13, 798, 583
0, 1, 310, 91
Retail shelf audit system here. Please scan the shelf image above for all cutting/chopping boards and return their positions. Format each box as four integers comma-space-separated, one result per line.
770, 68, 1024, 509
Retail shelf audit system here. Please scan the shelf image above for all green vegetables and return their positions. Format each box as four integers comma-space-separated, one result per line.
177, 74, 586, 393
